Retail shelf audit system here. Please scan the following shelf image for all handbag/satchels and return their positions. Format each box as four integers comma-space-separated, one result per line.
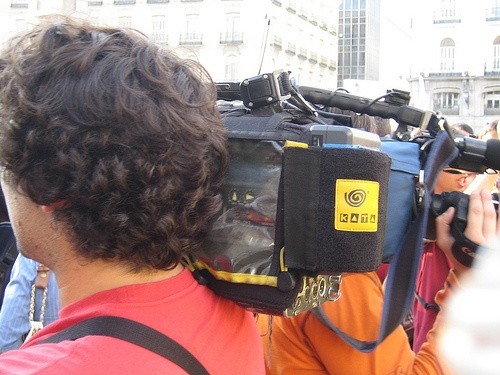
22, 319, 44, 345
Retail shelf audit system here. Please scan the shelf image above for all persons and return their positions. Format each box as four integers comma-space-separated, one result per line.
0, 15, 500, 373
